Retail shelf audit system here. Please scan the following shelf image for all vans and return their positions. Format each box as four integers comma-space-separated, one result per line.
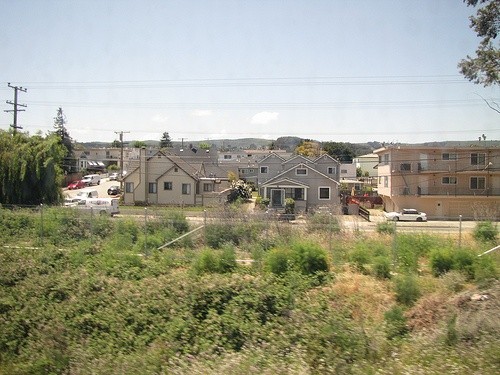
77, 191, 98, 198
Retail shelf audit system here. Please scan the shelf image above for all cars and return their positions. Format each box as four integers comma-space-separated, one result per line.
64, 198, 78, 206
107, 185, 119, 195
386, 208, 427, 222
67, 179, 85, 190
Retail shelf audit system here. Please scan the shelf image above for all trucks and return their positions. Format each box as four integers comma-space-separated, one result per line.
81, 174, 100, 186
74, 198, 120, 216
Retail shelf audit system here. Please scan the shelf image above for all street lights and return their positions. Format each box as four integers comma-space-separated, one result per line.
392, 169, 408, 190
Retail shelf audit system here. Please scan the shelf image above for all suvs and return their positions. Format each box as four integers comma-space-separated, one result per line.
109, 172, 118, 180
